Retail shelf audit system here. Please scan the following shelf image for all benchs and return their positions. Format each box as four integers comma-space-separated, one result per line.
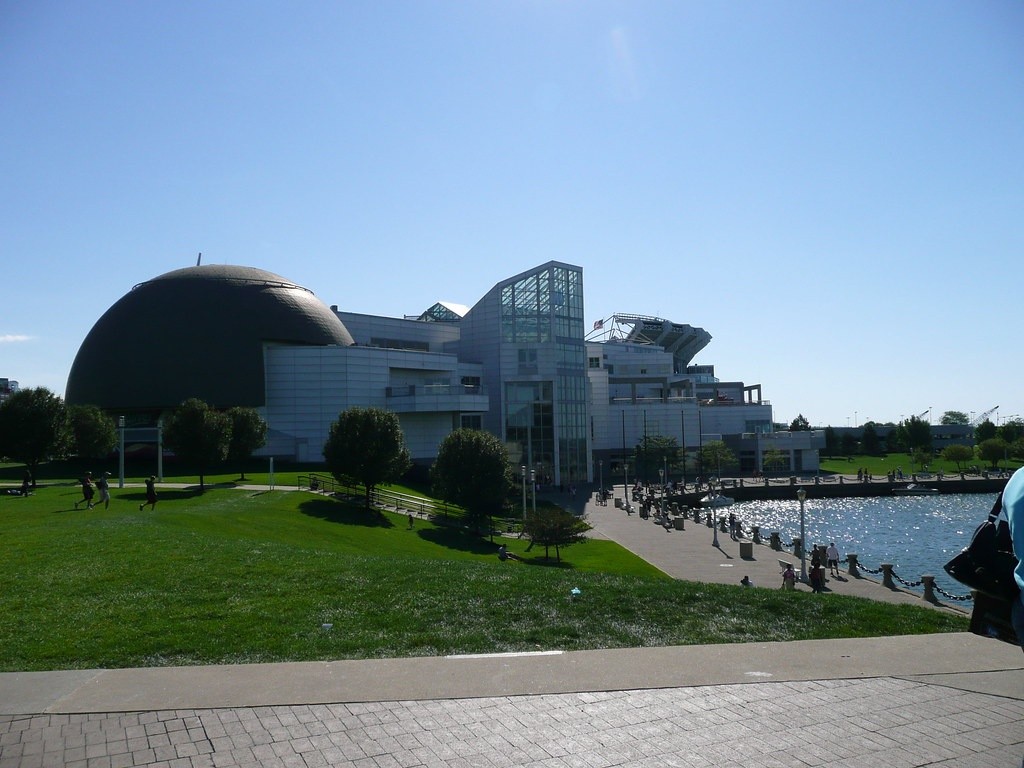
778, 559, 801, 583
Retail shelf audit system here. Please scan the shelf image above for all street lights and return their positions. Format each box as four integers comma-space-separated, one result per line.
710, 474, 719, 546
664, 456, 667, 485
797, 487, 808, 582
599, 459, 603, 492
531, 469, 536, 514
522, 466, 526, 520
659, 469, 665, 517
624, 463, 628, 502
929, 407, 932, 424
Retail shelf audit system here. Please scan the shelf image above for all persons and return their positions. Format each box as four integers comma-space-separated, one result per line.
826, 542, 840, 575
1001, 465, 1024, 649
742, 576, 753, 585
20, 470, 30, 497
497, 543, 511, 559
568, 486, 576, 499
74, 471, 112, 511
752, 469, 763, 481
810, 563, 823, 594
810, 544, 820, 566
140, 476, 157, 511
887, 466, 902, 479
729, 516, 736, 539
408, 514, 414, 528
857, 467, 867, 480
600, 474, 703, 503
782, 564, 795, 590
310, 477, 319, 490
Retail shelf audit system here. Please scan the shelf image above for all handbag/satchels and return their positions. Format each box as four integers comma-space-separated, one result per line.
942, 518, 1021, 600
967, 589, 1022, 647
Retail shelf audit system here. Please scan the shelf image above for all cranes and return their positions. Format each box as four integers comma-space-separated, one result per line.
917, 410, 929, 420
969, 405, 999, 427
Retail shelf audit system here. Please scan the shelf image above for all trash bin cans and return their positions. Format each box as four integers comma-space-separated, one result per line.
677, 483, 682, 491
675, 518, 685, 530
639, 507, 644, 517
791, 476, 796, 483
740, 542, 752, 558
615, 498, 622, 507
811, 566, 826, 585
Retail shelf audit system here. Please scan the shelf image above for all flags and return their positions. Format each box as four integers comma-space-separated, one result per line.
594, 319, 603, 329
